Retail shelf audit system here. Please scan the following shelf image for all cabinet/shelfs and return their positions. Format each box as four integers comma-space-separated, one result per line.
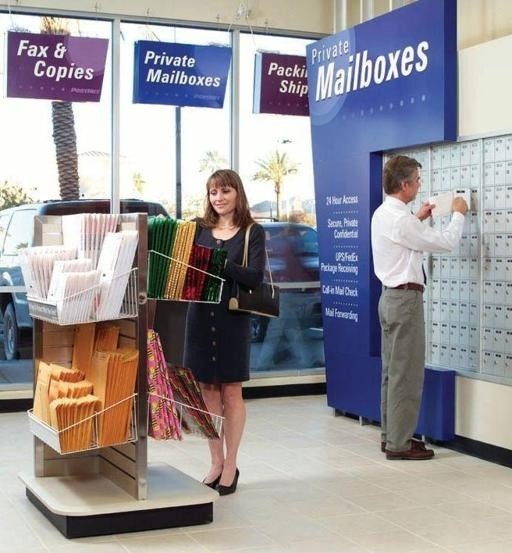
26, 213, 226, 539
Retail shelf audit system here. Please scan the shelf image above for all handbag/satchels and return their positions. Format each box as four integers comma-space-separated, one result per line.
227, 282, 279, 318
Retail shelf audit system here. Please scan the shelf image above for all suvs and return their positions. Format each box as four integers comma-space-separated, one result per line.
247, 214, 323, 341
0, 197, 178, 366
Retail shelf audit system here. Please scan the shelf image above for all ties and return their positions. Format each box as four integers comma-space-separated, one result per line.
422, 264, 426, 285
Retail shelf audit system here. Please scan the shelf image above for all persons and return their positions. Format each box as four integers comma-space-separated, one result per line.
178, 167, 267, 495
370, 154, 469, 461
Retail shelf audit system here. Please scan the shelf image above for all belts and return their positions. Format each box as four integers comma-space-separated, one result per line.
388, 283, 425, 292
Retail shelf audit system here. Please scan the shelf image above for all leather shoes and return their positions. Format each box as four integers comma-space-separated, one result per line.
218, 467, 239, 495
385, 449, 433, 460
203, 474, 221, 489
381, 439, 425, 451
